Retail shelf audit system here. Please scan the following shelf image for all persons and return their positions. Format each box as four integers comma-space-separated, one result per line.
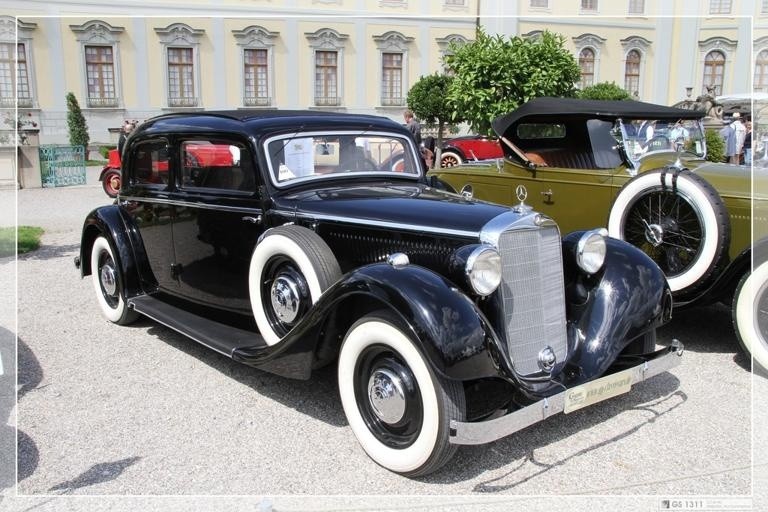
730, 113, 746, 166
671, 121, 691, 148
404, 107, 423, 147
741, 121, 752, 166
119, 123, 134, 158
636, 120, 654, 153
719, 116, 736, 163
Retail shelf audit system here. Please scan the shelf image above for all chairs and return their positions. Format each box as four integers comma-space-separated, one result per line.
198, 165, 261, 191
539, 145, 597, 170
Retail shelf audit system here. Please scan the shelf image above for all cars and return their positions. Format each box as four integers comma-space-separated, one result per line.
98, 139, 241, 197
73, 110, 683, 479
